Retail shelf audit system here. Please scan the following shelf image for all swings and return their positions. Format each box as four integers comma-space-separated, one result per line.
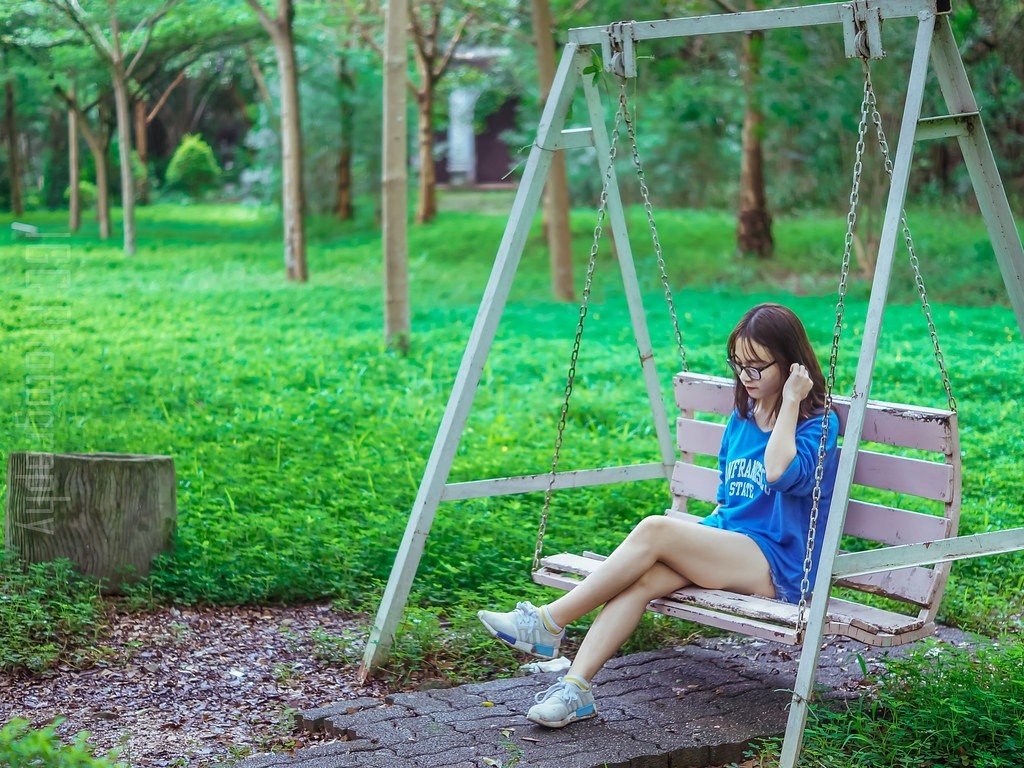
530, 27, 962, 645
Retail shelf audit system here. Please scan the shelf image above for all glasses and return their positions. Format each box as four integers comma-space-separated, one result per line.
726, 358, 778, 381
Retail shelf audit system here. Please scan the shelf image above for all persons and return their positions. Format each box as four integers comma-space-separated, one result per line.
478, 303, 840, 728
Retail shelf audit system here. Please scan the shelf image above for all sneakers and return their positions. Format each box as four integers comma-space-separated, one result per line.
526, 677, 597, 727
477, 601, 565, 659
